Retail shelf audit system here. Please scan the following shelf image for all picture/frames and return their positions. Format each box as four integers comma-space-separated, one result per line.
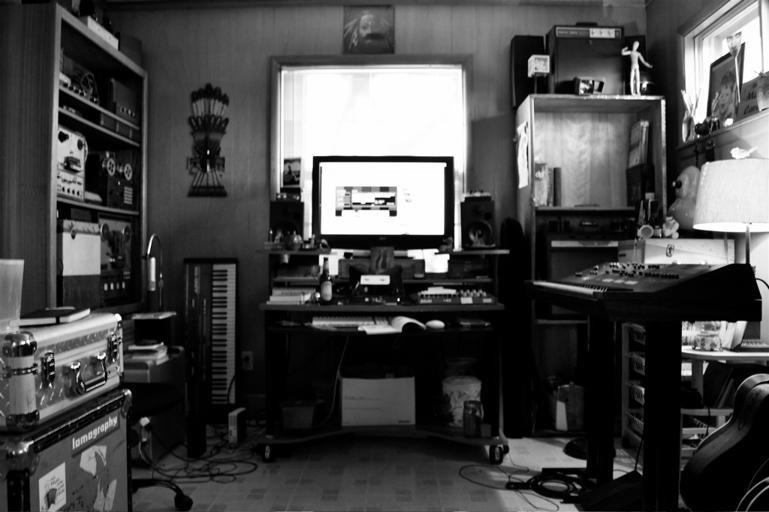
707, 43, 746, 121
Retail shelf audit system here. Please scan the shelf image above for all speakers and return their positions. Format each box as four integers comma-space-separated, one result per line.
510, 35, 544, 109
460, 199, 496, 249
269, 200, 303, 242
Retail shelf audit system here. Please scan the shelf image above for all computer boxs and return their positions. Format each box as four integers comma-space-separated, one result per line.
339, 258, 424, 279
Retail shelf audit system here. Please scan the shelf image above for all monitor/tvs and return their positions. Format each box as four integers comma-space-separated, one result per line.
312, 156, 454, 259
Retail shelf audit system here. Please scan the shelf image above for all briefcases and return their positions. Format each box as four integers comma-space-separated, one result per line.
0, 310, 122, 430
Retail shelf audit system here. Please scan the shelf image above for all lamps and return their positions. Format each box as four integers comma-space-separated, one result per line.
185, 80, 236, 199
692, 159, 769, 264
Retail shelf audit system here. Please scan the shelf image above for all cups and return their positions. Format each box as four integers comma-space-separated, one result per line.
0, 259, 24, 333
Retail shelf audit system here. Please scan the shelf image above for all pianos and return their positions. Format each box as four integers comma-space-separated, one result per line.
182, 257, 239, 425
530, 261, 762, 323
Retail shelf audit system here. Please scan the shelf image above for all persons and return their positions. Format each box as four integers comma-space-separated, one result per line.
344, 8, 393, 55
711, 72, 739, 122
726, 30, 744, 60
621, 41, 654, 96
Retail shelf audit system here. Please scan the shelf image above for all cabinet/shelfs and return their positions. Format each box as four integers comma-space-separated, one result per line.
617, 318, 769, 460
1, 1, 150, 320
512, 93, 671, 443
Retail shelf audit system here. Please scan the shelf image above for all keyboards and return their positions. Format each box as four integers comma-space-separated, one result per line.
312, 316, 389, 327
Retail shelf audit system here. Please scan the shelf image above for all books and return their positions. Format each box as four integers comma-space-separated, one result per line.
626, 165, 656, 207
627, 119, 649, 167
534, 162, 562, 208
20, 303, 93, 328
127, 342, 168, 360
270, 292, 311, 301
362, 315, 426, 335
267, 299, 308, 306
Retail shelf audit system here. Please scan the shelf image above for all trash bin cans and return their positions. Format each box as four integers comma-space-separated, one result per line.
441, 376, 482, 428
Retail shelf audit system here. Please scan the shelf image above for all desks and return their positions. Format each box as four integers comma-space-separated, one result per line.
251, 242, 509, 464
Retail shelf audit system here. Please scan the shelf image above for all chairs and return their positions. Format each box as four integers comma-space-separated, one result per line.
121, 327, 194, 511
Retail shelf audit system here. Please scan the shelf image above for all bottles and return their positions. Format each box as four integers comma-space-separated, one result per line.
320, 257, 333, 304
463, 400, 484, 438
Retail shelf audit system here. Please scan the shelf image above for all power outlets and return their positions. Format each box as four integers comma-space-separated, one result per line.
242, 352, 254, 370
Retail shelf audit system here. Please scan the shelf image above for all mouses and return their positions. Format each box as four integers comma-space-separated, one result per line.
426, 320, 445, 328
